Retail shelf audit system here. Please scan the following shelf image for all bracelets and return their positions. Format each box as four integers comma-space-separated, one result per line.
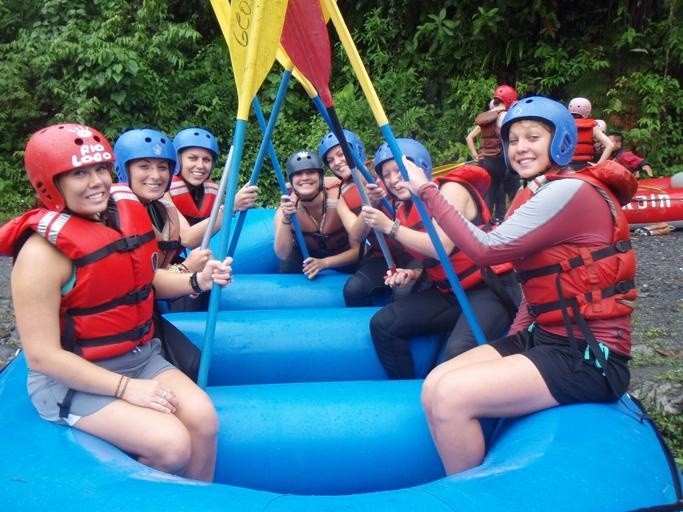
281, 221, 292, 225
190, 272, 203, 293
390, 219, 399, 238
114, 376, 125, 398
219, 206, 236, 217
169, 262, 190, 274
119, 378, 130, 398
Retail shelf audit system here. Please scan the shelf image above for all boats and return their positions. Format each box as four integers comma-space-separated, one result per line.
0, 206, 683, 511
619, 173, 683, 231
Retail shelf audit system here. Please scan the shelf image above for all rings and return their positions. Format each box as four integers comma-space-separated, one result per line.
162, 392, 166, 397
158, 398, 162, 403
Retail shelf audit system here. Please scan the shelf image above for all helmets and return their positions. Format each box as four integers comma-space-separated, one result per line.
501, 96, 577, 172
319, 129, 365, 179
173, 128, 218, 176
285, 151, 323, 193
114, 128, 176, 188
25, 123, 114, 210
569, 97, 593, 118
374, 138, 432, 198
494, 84, 519, 111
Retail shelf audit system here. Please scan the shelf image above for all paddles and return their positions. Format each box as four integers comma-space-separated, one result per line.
227, 63, 292, 257
322, 0, 487, 345
251, 95, 310, 259
275, 45, 398, 220
201, 135, 236, 248
198, 1, 290, 390
431, 158, 477, 178
281, 0, 398, 275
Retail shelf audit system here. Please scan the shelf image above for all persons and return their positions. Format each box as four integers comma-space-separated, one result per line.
317, 130, 420, 308
467, 85, 522, 222
162, 128, 258, 312
395, 97, 640, 478
567, 97, 652, 179
362, 140, 518, 379
1, 122, 233, 485
272, 150, 360, 279
116, 130, 212, 384
